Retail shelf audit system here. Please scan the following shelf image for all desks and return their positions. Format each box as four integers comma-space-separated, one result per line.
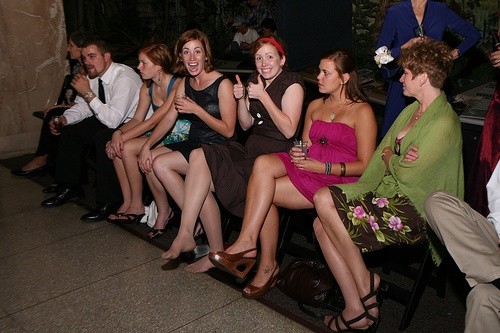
214, 59, 256, 73
452, 80, 497, 126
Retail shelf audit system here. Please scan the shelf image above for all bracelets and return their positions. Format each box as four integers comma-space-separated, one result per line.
326, 162, 328, 174
118, 129, 123, 134
340, 162, 345, 176
328, 162, 330, 174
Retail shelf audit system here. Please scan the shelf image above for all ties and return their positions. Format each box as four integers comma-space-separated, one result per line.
98, 78, 105, 104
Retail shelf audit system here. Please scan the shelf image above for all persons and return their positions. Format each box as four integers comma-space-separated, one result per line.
232, 0, 288, 63
313, 35, 464, 333
138, 29, 237, 239
482, 26, 500, 171
423, 158, 500, 333
160, 33, 305, 273
209, 47, 376, 298
105, 44, 182, 221
10, 31, 97, 185
374, 0, 481, 138
42, 40, 155, 221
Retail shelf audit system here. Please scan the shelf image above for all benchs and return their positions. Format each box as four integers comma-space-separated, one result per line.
300, 64, 388, 106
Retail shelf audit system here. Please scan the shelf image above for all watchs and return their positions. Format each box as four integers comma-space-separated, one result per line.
86, 92, 93, 97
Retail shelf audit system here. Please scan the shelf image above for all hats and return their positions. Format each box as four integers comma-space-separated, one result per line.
232, 16, 246, 26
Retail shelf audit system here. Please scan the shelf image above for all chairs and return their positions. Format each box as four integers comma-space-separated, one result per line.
33, 76, 449, 333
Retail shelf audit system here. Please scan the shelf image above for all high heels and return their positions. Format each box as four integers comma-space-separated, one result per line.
242, 261, 281, 298
147, 209, 177, 240
323, 311, 369, 333
107, 210, 146, 224
194, 228, 204, 240
361, 271, 389, 333
208, 248, 257, 279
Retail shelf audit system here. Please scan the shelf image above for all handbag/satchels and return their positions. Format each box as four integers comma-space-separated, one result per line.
276, 259, 334, 308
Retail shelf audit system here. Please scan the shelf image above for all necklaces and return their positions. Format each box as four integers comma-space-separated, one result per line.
416, 111, 424, 119
330, 99, 348, 121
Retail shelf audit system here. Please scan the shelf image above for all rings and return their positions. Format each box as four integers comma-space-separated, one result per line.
53, 116, 58, 120
301, 165, 302, 168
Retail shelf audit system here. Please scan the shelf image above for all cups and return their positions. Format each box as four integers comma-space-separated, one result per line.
294, 140, 308, 158
174, 94, 187, 99
52, 116, 64, 135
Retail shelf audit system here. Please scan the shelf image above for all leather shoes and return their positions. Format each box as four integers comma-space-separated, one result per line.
41, 186, 84, 208
80, 200, 121, 221
11, 156, 49, 176
42, 183, 65, 193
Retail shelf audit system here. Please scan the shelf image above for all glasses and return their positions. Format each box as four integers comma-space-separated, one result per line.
68, 44, 76, 49
